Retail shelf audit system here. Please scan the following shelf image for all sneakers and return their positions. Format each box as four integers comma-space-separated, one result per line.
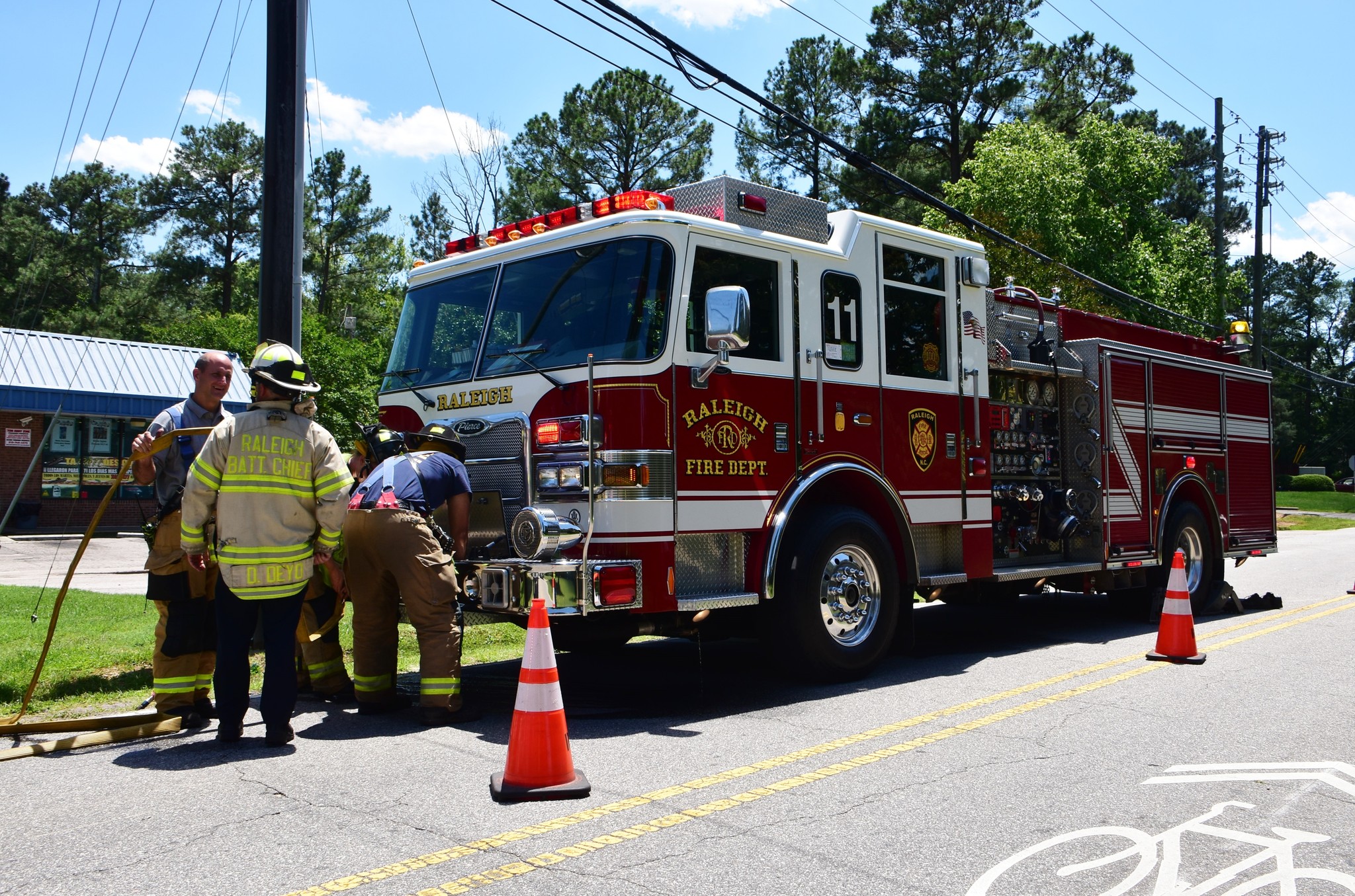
324, 682, 356, 704
195, 698, 220, 720
161, 704, 203, 729
297, 683, 321, 696
266, 723, 294, 747
216, 720, 245, 744
356, 695, 413, 716
416, 704, 483, 726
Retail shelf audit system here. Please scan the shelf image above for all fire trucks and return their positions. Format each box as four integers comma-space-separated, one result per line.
373, 174, 1279, 685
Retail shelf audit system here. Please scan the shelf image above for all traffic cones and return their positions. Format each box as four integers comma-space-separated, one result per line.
1145, 551, 1207, 665
488, 598, 592, 801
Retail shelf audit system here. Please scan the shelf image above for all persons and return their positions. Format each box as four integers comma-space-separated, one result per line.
293, 427, 405, 700
178, 339, 356, 744
129, 352, 235, 728
343, 423, 475, 726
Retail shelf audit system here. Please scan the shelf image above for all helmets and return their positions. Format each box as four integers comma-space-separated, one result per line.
242, 339, 320, 396
353, 421, 407, 466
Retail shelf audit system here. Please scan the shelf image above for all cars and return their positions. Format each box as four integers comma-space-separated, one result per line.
1334, 477, 1354, 493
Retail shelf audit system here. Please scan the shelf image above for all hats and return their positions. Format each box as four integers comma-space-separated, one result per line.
409, 423, 466, 466
